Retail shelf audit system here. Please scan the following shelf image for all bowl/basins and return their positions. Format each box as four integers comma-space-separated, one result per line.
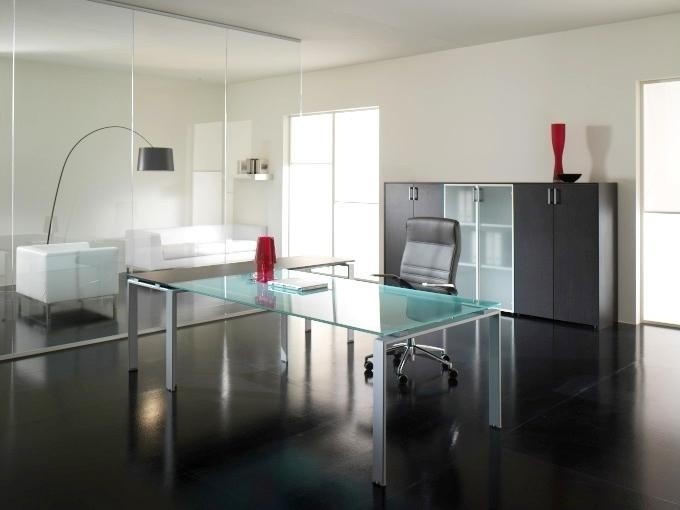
559, 175, 583, 183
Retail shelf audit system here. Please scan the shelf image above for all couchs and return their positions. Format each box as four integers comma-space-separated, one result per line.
125, 222, 268, 283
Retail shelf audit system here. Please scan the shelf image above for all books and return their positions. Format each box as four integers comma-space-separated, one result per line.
269, 277, 330, 292
456, 189, 466, 222
466, 190, 474, 223
493, 230, 507, 267
468, 228, 476, 263
483, 229, 493, 266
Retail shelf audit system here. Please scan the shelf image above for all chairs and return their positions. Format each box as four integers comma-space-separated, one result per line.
17, 243, 121, 332
361, 217, 463, 394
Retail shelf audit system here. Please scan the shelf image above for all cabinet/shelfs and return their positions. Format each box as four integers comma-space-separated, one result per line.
385, 183, 444, 289
511, 183, 618, 332
444, 184, 513, 314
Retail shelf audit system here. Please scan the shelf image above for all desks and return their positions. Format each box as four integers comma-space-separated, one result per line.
124, 253, 503, 493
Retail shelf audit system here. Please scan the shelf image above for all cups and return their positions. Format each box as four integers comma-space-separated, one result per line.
255, 235, 276, 283
235, 157, 269, 175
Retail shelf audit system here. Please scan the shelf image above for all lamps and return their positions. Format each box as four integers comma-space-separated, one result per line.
46, 123, 176, 247
